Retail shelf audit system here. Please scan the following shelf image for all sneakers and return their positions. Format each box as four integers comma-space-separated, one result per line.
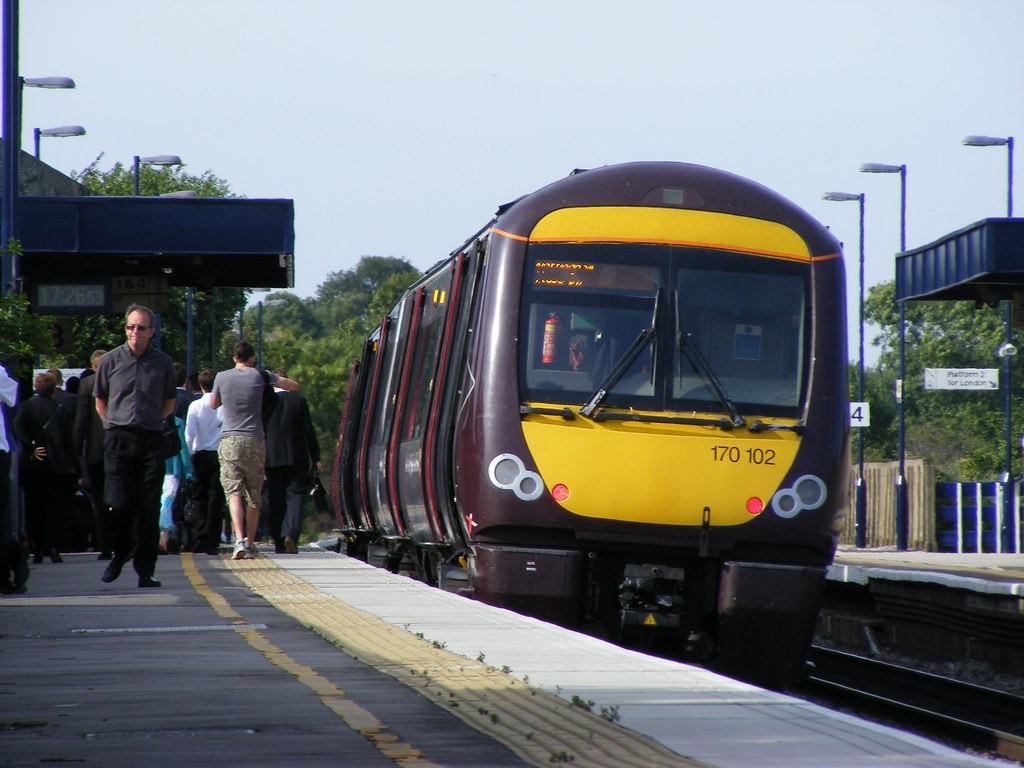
232, 542, 247, 559
243, 545, 258, 558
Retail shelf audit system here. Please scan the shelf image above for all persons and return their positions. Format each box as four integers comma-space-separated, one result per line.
185, 369, 222, 555
570, 336, 584, 371
92, 305, 177, 588
211, 342, 298, 559
75, 345, 202, 559
377, 452, 422, 506
0, 365, 29, 595
14, 368, 79, 563
265, 368, 322, 553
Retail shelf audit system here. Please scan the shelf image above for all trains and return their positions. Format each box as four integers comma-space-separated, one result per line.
334, 162, 846, 686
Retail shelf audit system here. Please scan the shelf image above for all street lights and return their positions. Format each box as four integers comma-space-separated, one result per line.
960, 133, 1015, 554
819, 191, 868, 549
131, 153, 182, 196
14, 76, 85, 196
858, 161, 908, 553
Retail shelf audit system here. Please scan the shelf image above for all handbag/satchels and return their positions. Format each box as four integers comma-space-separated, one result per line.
310, 470, 330, 510
177, 480, 201, 524
256, 367, 279, 409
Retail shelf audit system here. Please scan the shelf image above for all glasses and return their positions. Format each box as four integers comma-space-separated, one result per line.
126, 325, 149, 331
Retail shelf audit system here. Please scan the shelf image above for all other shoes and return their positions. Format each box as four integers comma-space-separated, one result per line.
139, 578, 161, 587
50, 548, 63, 563
285, 536, 297, 553
33, 555, 43, 563
276, 546, 284, 553
103, 554, 131, 583
